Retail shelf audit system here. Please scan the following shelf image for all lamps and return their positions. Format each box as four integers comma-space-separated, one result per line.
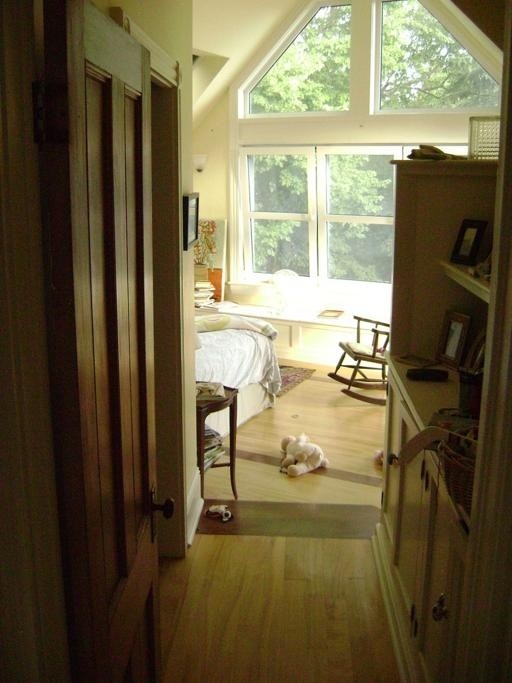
192, 153, 209, 173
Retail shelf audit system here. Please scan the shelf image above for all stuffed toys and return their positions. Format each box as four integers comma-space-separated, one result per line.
280, 434, 329, 477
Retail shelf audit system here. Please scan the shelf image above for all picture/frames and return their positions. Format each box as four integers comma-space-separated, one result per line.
451, 218, 489, 266
182, 191, 200, 251
434, 310, 472, 368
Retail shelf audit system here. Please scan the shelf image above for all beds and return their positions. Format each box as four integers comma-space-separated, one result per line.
193, 306, 283, 439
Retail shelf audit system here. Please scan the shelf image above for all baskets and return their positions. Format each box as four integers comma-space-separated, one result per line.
437, 426, 479, 505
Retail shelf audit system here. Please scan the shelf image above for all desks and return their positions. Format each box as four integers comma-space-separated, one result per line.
196, 385, 240, 500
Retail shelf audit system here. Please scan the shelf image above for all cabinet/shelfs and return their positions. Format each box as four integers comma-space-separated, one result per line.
370, 370, 470, 682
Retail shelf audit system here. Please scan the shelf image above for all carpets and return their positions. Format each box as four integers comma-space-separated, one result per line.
273, 365, 317, 398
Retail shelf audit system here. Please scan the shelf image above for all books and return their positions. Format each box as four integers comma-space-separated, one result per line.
204, 423, 226, 471
194, 280, 216, 307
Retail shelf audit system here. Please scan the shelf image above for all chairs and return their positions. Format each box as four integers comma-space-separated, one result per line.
326, 315, 391, 406
